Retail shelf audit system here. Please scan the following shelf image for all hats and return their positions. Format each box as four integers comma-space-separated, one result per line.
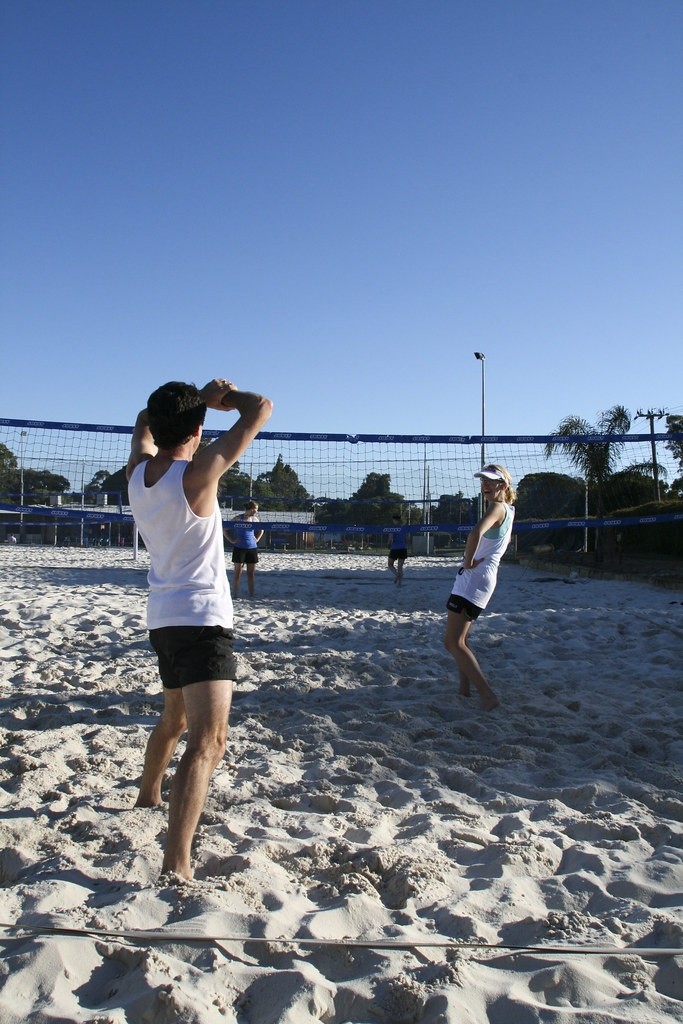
473, 467, 509, 486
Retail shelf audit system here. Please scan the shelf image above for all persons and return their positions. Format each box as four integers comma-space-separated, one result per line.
63, 532, 109, 547
444, 465, 517, 711
8, 533, 17, 544
126, 377, 273, 882
119, 535, 125, 546
386, 513, 407, 585
223, 501, 264, 598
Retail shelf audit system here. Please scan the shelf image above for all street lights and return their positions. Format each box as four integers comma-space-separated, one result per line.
20, 431, 27, 543
474, 352, 487, 516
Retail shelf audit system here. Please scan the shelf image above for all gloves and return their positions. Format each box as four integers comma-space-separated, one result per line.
205, 379, 238, 411
